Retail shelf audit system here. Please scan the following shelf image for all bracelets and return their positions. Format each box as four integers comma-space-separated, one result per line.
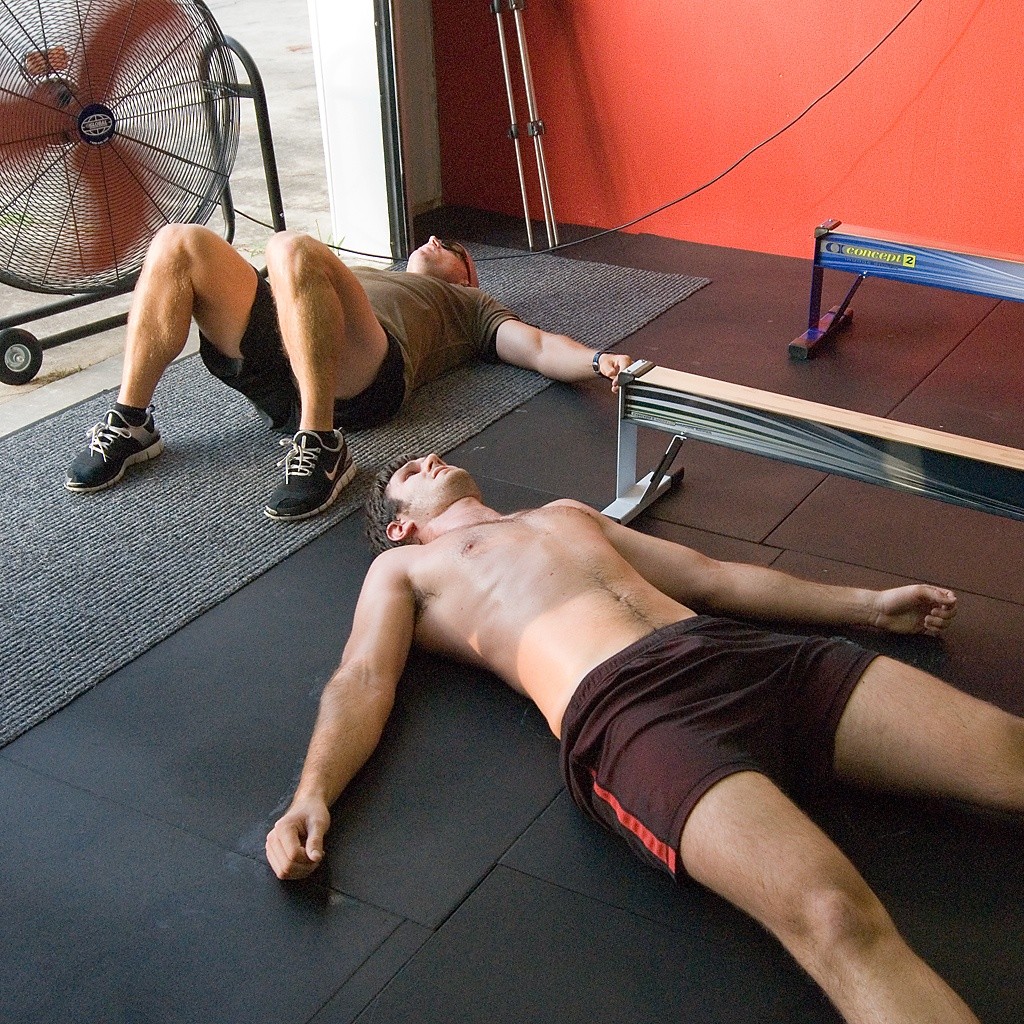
594, 349, 619, 380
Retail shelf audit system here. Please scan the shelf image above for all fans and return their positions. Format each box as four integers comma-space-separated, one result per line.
0, 1, 241, 293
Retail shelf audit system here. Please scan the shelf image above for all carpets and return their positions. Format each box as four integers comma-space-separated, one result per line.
0, 243, 706, 744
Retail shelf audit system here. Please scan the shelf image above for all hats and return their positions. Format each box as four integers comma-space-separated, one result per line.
455, 242, 480, 287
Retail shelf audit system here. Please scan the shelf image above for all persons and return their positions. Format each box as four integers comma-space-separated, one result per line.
266, 448, 1024, 1022
63, 217, 644, 521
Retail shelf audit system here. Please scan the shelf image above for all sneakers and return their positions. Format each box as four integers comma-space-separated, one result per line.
66, 404, 163, 491
263, 426, 354, 520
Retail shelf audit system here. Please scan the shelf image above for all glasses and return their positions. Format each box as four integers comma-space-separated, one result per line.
439, 239, 471, 284
